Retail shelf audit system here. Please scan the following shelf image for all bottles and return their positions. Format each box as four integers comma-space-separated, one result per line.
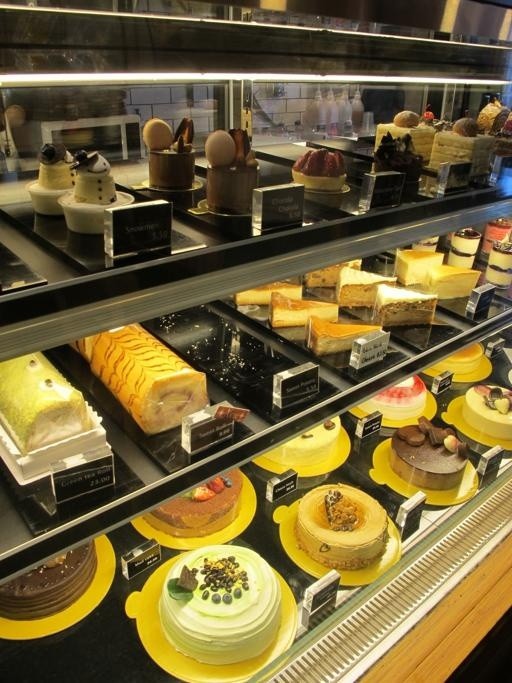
338, 85, 352, 135
350, 86, 364, 132
310, 85, 326, 139
325, 86, 338, 138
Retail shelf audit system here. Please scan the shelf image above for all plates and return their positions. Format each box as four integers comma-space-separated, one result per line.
130, 176, 203, 192
273, 485, 402, 588
1, 532, 118, 641
188, 197, 253, 217
421, 353, 494, 384
0, 449, 146, 537
254, 142, 483, 199
348, 388, 438, 430
131, 469, 258, 550
441, 395, 512, 451
0, 198, 210, 277
218, 282, 411, 385
46, 350, 256, 474
369, 437, 479, 507
141, 305, 340, 426
302, 184, 352, 194
304, 268, 465, 356
124, 545, 297, 683
115, 168, 329, 238
436, 294, 512, 325
253, 425, 351, 480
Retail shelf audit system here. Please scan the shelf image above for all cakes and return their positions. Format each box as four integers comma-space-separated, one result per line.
374, 285, 437, 326
305, 316, 382, 354
268, 291, 338, 328
143, 469, 245, 537
336, 267, 396, 307
421, 109, 454, 133
28, 142, 76, 216
430, 118, 495, 176
447, 229, 481, 268
143, 116, 195, 189
305, 260, 361, 287
71, 322, 209, 435
463, 383, 512, 442
292, 148, 347, 190
204, 129, 259, 213
484, 241, 512, 290
358, 375, 426, 420
483, 219, 512, 254
374, 111, 435, 165
0, 351, 90, 453
430, 343, 482, 375
388, 417, 470, 490
413, 236, 438, 252
395, 250, 444, 284
0, 539, 97, 620
236, 282, 302, 305
159, 545, 282, 664
477, 102, 512, 155
421, 263, 481, 299
58, 150, 134, 235
295, 484, 389, 570
262, 415, 341, 467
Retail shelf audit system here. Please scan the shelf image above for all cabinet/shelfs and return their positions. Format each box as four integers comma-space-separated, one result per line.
0, 2, 511, 683
